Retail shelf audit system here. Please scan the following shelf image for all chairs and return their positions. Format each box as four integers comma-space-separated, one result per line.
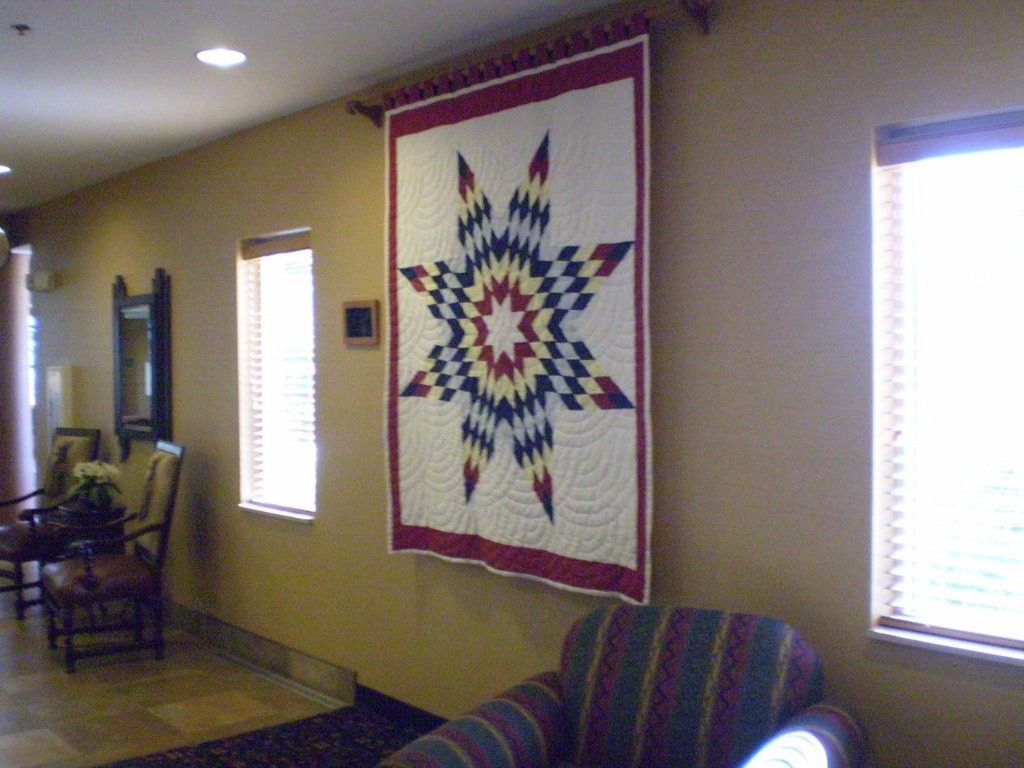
0, 424, 185, 673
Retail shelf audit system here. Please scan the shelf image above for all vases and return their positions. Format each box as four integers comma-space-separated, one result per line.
90, 484, 113, 510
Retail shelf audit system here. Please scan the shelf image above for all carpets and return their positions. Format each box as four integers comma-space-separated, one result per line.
88, 705, 440, 768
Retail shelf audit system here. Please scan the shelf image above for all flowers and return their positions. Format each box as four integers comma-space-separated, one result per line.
69, 460, 124, 487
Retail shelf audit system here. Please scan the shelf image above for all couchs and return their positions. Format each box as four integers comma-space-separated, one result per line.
382, 601, 877, 768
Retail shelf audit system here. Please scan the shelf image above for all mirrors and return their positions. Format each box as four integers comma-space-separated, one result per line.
110, 268, 173, 459
